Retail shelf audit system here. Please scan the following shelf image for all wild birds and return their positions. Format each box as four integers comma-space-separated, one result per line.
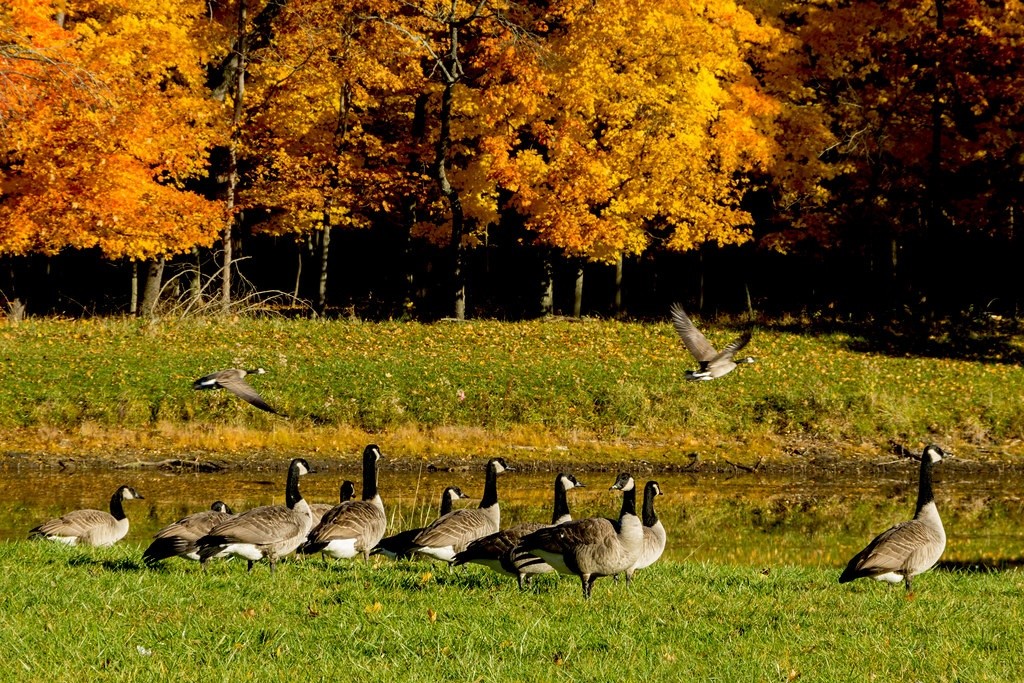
190, 366, 293, 420
838, 444, 949, 592
669, 300, 763, 383
31, 441, 670, 603
